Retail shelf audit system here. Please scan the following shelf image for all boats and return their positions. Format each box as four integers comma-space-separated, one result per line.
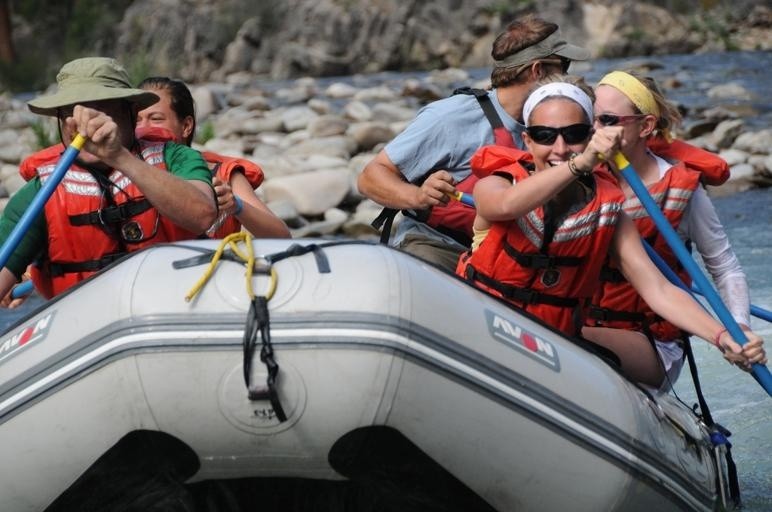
1, 235, 739, 509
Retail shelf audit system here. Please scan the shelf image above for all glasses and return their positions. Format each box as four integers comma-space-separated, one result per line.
526, 123, 591, 145
599, 115, 645, 125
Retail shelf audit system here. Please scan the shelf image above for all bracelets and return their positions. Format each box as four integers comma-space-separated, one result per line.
716, 327, 728, 347
230, 195, 243, 215
568, 152, 593, 177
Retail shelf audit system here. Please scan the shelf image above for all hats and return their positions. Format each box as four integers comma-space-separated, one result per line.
494, 25, 589, 68
27, 57, 160, 116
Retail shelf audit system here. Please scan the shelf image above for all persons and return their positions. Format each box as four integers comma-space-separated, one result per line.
357, 13, 592, 278
454, 72, 768, 372
580, 69, 755, 393
0, 56, 219, 304
0, 77, 292, 310
